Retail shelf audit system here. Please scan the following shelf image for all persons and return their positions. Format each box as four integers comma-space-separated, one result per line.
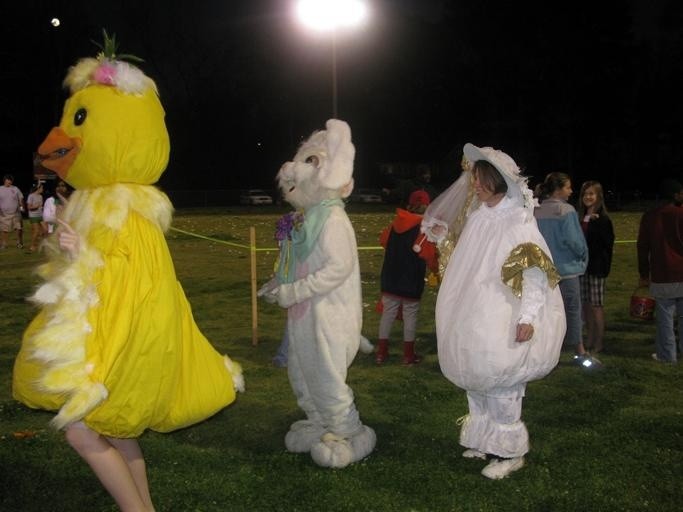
10, 27, 247, 511
0, 174, 25, 251
531, 171, 603, 367
377, 188, 440, 365
26, 178, 46, 253
636, 178, 682, 362
42, 179, 72, 234
431, 140, 568, 481
576, 179, 616, 355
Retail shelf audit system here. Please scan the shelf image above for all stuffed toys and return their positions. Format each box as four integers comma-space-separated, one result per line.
257, 117, 378, 469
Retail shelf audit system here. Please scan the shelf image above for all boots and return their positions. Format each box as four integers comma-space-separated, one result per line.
399, 340, 423, 365
373, 338, 390, 366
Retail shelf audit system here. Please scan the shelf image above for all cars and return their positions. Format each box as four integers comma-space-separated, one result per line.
350, 187, 382, 203
235, 188, 275, 205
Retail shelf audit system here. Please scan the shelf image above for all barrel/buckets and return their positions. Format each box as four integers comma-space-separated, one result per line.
630, 286, 656, 323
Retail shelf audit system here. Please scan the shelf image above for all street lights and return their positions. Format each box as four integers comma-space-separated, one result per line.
297, 0, 373, 120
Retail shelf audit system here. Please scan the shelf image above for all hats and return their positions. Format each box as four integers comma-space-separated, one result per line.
407, 188, 431, 207
462, 138, 522, 197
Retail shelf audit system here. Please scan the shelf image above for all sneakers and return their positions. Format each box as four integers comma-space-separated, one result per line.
478, 455, 527, 481
461, 448, 488, 461
576, 351, 603, 370
650, 352, 665, 362
